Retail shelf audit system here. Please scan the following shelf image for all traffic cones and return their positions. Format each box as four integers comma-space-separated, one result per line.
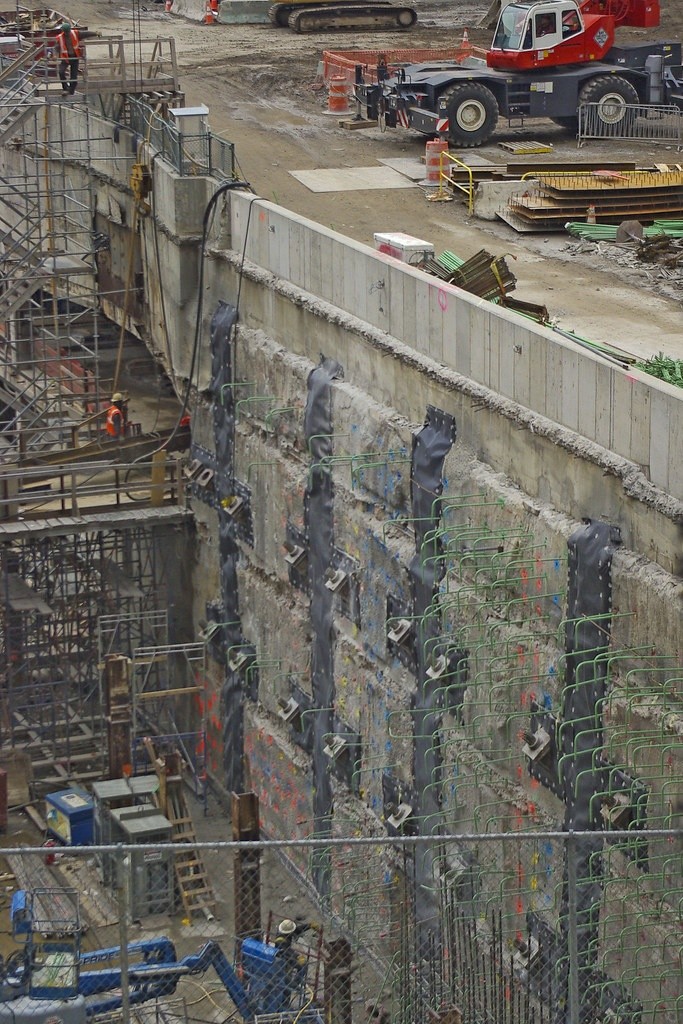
204, 3, 215, 24
163, 0, 172, 13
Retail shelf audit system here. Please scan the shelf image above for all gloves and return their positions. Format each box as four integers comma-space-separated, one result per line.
310, 921, 319, 930
298, 956, 305, 962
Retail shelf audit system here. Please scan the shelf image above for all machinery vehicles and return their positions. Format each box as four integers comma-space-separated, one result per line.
352, 0, 683, 150
269, 0, 417, 34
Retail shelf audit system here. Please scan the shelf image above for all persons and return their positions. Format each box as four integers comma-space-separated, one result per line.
106, 393, 127, 440
536, 14, 556, 37
47, 23, 103, 97
275, 919, 319, 967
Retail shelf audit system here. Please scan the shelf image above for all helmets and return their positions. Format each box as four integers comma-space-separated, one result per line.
61, 23, 71, 31
111, 393, 123, 402
279, 919, 296, 933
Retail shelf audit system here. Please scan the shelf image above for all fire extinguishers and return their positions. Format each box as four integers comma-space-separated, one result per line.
39, 839, 55, 864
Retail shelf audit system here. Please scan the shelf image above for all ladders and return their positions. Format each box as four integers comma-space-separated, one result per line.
163, 792, 222, 927
